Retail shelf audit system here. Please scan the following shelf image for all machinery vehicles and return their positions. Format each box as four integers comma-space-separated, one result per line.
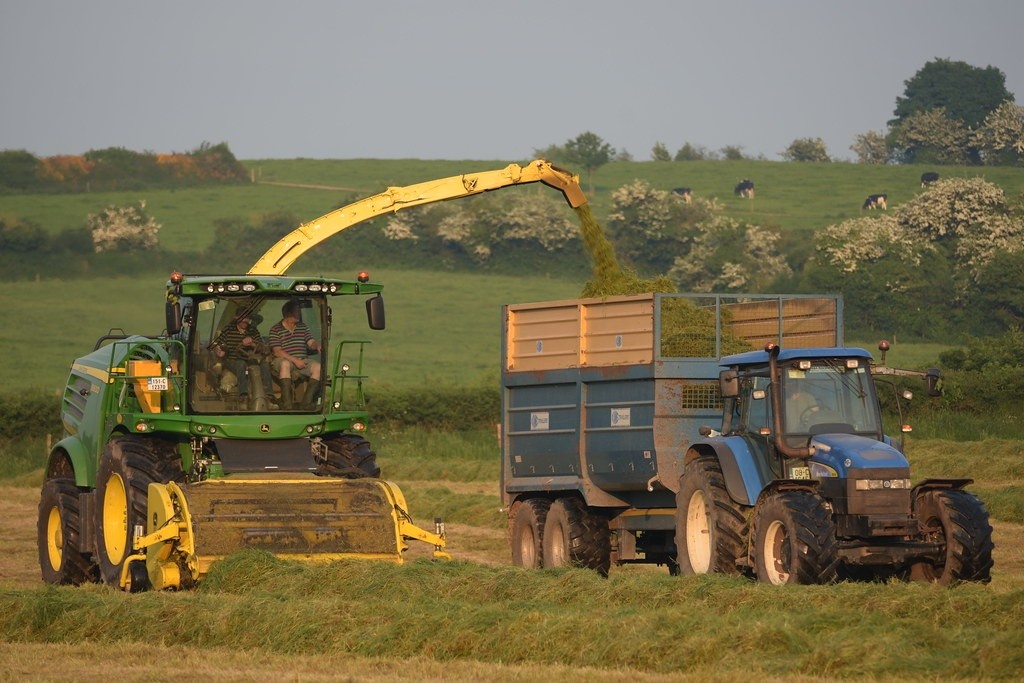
497, 293, 993, 589
37, 158, 589, 593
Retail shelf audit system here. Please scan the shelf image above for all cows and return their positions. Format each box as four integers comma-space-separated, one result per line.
671, 188, 694, 204
862, 193, 887, 210
735, 180, 753, 199
920, 172, 943, 189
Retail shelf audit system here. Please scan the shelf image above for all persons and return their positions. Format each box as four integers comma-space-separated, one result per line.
785, 383, 819, 433
269, 302, 321, 411
214, 308, 280, 411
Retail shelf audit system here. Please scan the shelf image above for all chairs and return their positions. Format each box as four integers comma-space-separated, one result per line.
204, 330, 309, 410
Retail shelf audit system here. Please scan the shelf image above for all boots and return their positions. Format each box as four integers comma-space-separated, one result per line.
301, 378, 320, 410
279, 378, 292, 409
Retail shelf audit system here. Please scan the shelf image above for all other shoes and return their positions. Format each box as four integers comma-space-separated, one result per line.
239, 395, 248, 410
268, 396, 280, 410
